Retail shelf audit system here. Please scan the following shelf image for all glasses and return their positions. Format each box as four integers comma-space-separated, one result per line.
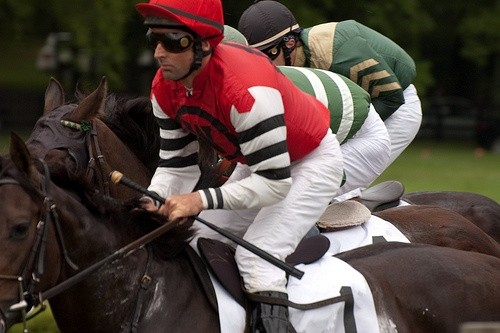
145, 30, 195, 53
257, 36, 294, 61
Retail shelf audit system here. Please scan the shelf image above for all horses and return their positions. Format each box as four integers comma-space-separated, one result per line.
0, 74, 500, 332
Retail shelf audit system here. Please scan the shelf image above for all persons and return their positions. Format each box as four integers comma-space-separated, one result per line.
131, 1, 422, 332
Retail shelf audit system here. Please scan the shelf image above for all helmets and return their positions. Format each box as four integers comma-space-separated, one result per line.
136, 0, 225, 49
237, 1, 302, 52
